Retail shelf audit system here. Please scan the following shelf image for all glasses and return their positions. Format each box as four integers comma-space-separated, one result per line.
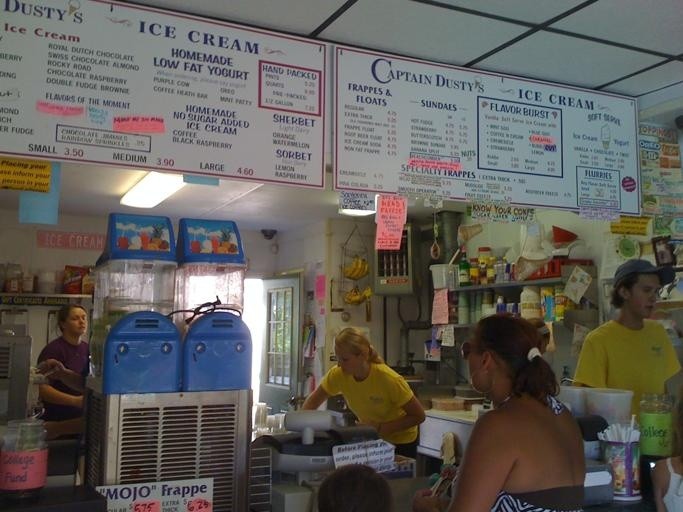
461, 341, 474, 361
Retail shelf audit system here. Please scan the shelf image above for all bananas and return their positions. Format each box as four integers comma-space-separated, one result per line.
343, 254, 369, 280
344, 285, 373, 322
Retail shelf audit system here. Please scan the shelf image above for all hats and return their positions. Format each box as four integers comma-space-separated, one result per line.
613, 258, 676, 288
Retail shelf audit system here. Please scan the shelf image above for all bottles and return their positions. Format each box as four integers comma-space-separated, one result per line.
459, 246, 516, 287
638, 392, 674, 460
457, 284, 543, 325
0, 418, 50, 500
0, 259, 23, 293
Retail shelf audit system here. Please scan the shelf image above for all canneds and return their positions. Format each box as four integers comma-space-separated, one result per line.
5, 263, 24, 293
540, 283, 589, 322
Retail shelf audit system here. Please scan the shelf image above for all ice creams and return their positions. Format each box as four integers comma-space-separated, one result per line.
601, 123, 611, 150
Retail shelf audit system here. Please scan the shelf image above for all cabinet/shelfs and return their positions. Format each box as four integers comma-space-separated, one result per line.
432, 265, 600, 331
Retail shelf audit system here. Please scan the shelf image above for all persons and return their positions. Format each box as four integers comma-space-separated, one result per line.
301, 326, 426, 458
37, 303, 89, 441
651, 394, 683, 512
411, 311, 587, 512
318, 464, 393, 512
572, 259, 683, 421
